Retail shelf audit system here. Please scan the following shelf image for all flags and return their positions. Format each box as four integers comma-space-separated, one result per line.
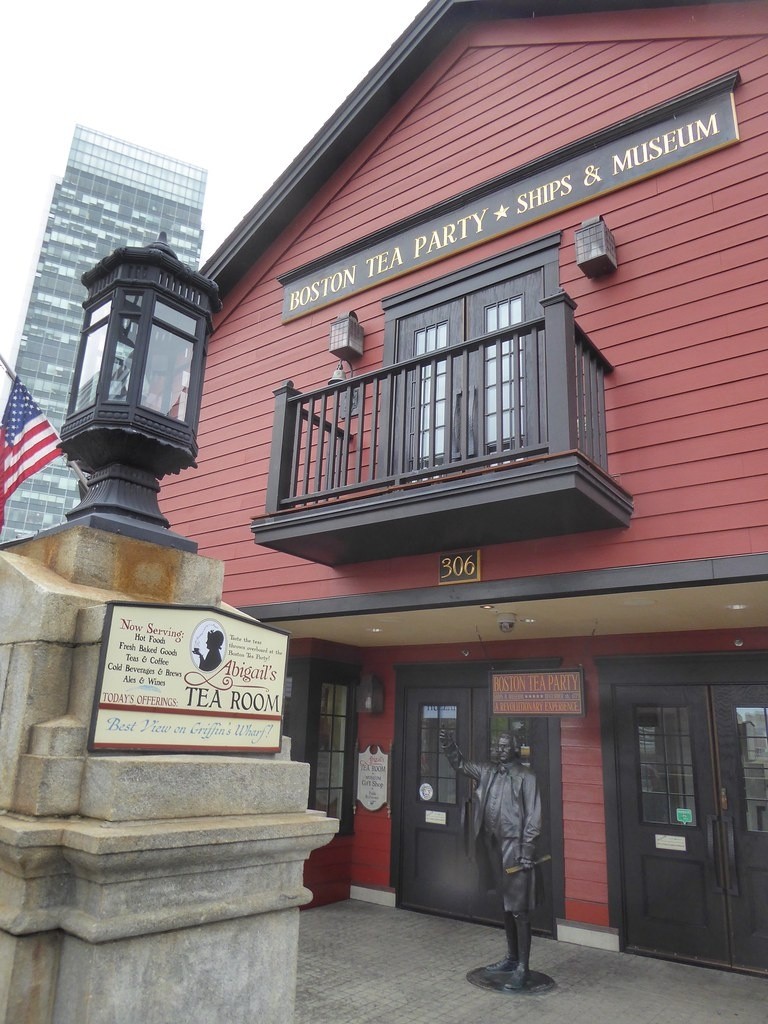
0, 375, 62, 533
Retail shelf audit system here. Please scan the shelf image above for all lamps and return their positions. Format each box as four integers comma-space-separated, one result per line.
573, 213, 621, 282
61, 232, 214, 547
330, 312, 364, 363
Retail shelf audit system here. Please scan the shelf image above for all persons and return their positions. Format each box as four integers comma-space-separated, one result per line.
439, 724, 545, 990
